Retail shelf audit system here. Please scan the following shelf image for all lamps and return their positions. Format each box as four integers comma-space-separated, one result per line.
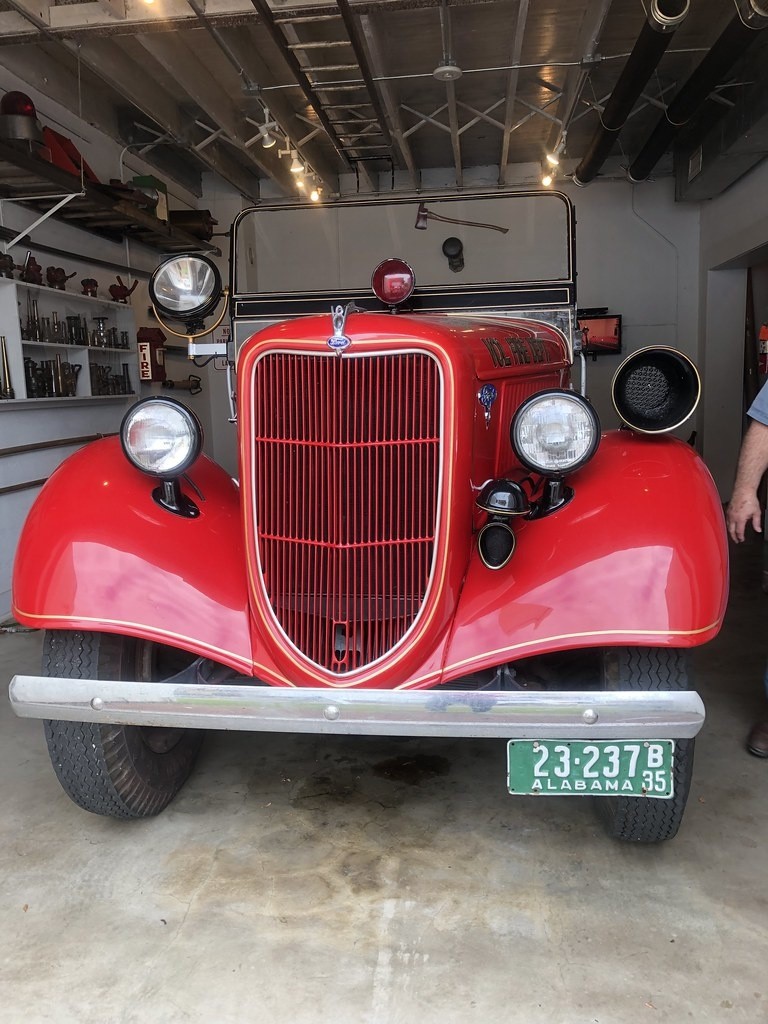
545, 143, 566, 165
278, 136, 305, 173
258, 108, 276, 148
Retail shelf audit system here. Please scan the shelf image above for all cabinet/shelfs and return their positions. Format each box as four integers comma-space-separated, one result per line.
0, 276, 142, 404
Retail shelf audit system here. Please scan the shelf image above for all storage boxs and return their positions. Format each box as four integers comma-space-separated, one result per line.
133, 174, 168, 222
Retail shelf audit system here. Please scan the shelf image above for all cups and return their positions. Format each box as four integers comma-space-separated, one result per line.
40, 359, 59, 397
66, 316, 81, 345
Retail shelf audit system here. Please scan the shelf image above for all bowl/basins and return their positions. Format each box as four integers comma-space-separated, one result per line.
0, 114, 47, 150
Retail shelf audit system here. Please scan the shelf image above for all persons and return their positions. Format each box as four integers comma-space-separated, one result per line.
725, 375, 768, 759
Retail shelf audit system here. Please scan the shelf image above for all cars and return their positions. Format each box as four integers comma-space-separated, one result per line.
8, 186, 731, 847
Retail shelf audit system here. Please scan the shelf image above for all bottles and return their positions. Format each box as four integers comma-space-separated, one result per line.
121, 331, 129, 348
92, 319, 109, 347
109, 327, 118, 348
41, 317, 54, 343
